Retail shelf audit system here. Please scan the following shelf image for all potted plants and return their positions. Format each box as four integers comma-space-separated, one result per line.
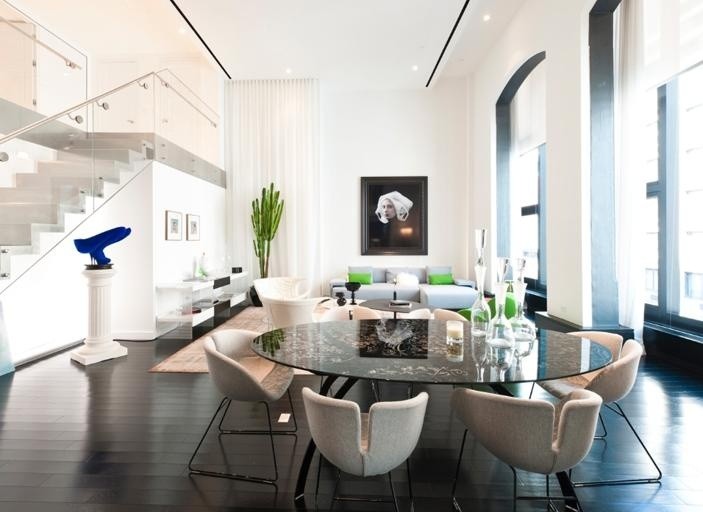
247, 182, 285, 307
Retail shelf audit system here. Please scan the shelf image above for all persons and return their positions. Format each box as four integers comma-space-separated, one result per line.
373, 190, 415, 248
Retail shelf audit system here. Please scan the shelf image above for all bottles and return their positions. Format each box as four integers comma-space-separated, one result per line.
511, 281, 537, 342
485, 281, 515, 348
514, 256, 527, 280
471, 336, 534, 383
471, 264, 490, 337
495, 257, 509, 282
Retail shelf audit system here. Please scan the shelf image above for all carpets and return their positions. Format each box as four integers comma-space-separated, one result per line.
141, 304, 316, 375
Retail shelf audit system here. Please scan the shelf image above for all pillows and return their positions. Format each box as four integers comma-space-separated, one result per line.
347, 271, 372, 284
427, 272, 452, 285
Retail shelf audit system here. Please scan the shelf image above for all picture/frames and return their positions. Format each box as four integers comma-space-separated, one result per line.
166, 209, 201, 241
360, 177, 428, 255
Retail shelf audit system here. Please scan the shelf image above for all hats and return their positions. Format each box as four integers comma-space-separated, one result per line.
374, 191, 413, 224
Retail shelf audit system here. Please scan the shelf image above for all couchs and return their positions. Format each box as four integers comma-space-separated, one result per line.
330, 265, 477, 309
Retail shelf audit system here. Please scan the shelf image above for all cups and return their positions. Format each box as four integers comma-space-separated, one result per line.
446, 342, 463, 363
446, 321, 463, 345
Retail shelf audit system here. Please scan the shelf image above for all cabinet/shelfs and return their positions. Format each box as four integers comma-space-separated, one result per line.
157, 272, 247, 326
